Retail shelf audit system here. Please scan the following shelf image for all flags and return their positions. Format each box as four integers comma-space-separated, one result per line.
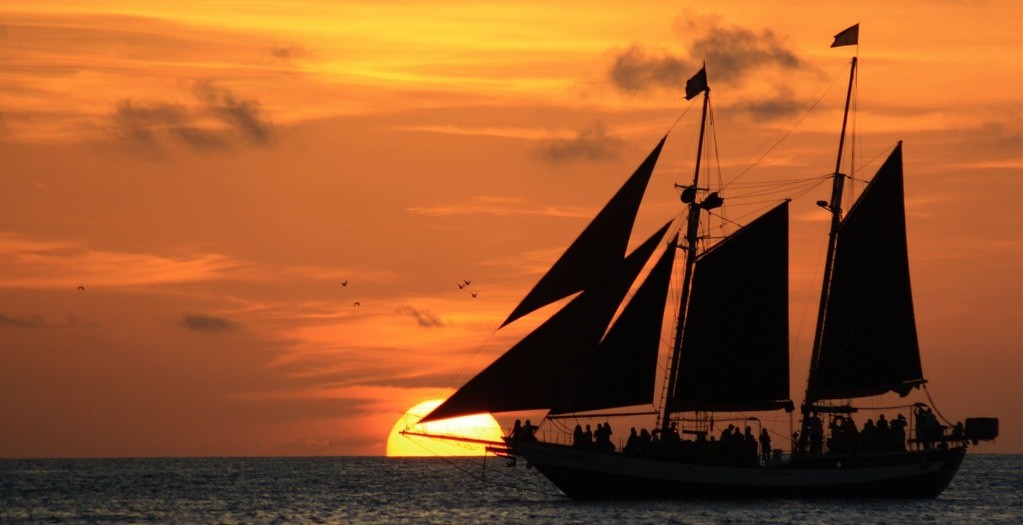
833, 23, 859, 47
685, 70, 706, 99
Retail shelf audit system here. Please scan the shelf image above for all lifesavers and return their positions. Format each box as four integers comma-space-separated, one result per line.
834, 430, 869, 466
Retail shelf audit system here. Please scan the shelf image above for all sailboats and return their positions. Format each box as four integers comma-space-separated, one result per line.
399, 21, 998, 505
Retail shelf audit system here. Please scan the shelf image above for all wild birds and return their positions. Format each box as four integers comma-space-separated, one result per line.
457, 279, 479, 298
76, 285, 84, 291
341, 280, 360, 307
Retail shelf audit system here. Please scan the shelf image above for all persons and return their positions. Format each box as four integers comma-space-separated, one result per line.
512, 419, 540, 443
575, 406, 941, 463
